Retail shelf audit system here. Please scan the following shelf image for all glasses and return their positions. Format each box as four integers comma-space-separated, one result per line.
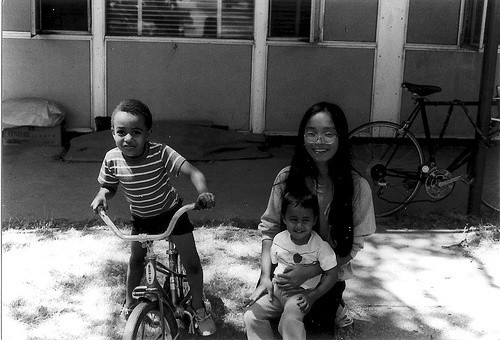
304, 131, 339, 145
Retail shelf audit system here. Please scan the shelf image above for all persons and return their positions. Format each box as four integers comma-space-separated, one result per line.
243, 101, 377, 339
89, 98, 218, 336
243, 188, 338, 339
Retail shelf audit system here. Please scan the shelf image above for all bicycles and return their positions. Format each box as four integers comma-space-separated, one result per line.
91, 196, 205, 340
340, 81, 500, 220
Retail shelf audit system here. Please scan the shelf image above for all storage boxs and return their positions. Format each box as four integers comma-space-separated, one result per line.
2, 125, 61, 146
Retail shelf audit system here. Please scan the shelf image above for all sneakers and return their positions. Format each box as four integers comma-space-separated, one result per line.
118, 299, 137, 321
190, 298, 216, 337
